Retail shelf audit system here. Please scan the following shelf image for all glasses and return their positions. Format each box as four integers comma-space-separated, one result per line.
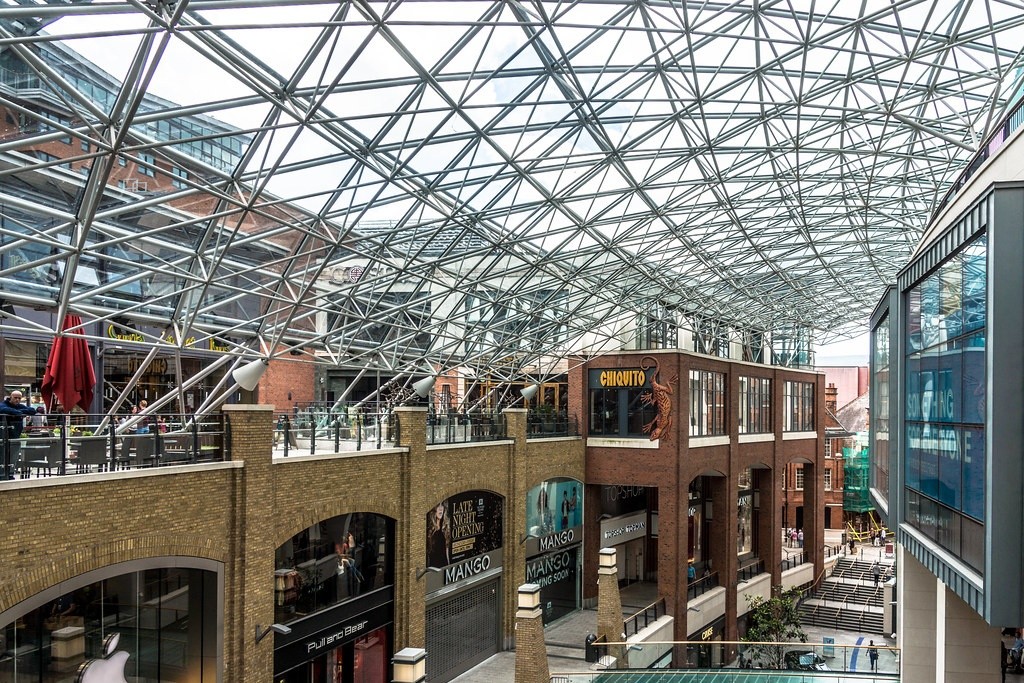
14, 396, 21, 399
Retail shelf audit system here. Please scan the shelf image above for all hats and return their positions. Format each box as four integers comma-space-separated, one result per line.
882, 527, 884, 530
798, 529, 801, 532
870, 528, 874, 531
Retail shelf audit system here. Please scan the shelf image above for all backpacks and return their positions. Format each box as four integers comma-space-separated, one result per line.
277, 420, 284, 430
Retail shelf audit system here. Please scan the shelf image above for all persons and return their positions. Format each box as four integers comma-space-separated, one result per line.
687, 562, 696, 591
865, 640, 878, 670
1002, 631, 1024, 683
427, 498, 450, 567
734, 652, 754, 668
50, 592, 77, 616
885, 565, 892, 581
334, 529, 355, 599
0, 390, 168, 480
536, 486, 577, 535
787, 529, 804, 548
849, 535, 856, 555
869, 527, 886, 547
873, 562, 880, 586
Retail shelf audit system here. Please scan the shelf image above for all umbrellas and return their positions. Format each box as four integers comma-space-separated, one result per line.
41, 313, 97, 470
